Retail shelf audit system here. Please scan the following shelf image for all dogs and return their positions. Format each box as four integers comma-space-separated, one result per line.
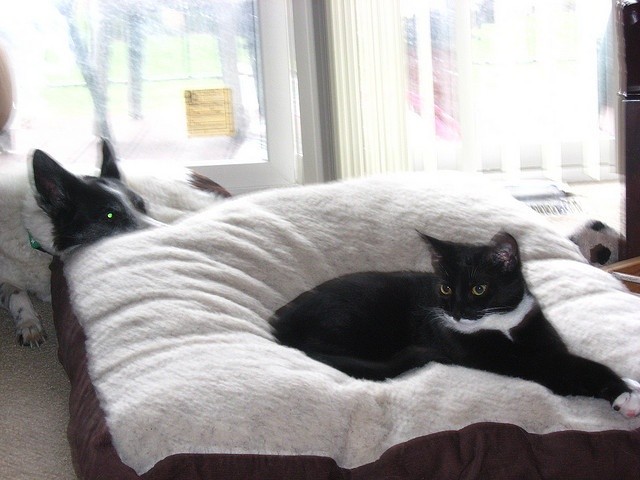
1, 136, 233, 350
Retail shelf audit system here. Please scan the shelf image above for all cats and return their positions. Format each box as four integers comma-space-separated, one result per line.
270, 228, 639, 419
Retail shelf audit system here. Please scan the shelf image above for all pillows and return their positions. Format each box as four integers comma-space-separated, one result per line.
49, 175, 640, 480
